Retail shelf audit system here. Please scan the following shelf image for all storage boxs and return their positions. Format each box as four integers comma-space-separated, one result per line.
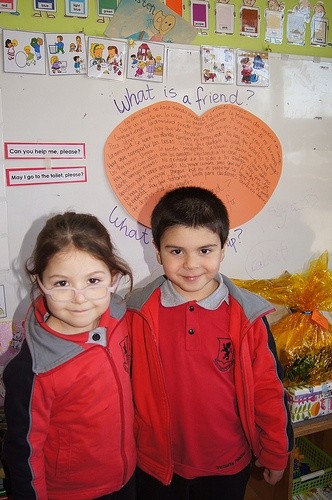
283, 382, 332, 423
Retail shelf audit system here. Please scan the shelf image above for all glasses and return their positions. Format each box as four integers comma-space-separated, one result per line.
37, 272, 122, 303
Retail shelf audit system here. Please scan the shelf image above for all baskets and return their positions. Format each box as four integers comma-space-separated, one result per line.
292, 436, 332, 494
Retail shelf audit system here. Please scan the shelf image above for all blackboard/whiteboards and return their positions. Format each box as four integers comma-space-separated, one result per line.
0, 30, 332, 359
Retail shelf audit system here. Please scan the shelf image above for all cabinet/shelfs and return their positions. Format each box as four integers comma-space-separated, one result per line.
241, 413, 332, 500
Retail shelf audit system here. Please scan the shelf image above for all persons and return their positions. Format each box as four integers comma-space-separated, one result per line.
0, 212, 137, 500
125, 187, 295, 500
